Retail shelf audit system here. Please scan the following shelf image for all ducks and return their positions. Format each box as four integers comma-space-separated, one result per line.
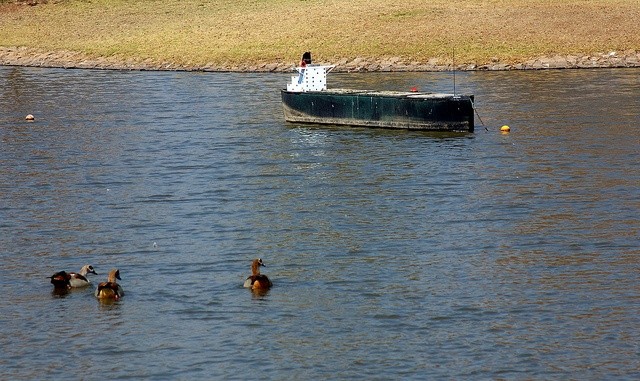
45, 264, 97, 290
96, 268, 124, 302
242, 260, 273, 290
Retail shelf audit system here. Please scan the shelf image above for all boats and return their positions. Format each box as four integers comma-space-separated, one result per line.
276, 50, 475, 133
